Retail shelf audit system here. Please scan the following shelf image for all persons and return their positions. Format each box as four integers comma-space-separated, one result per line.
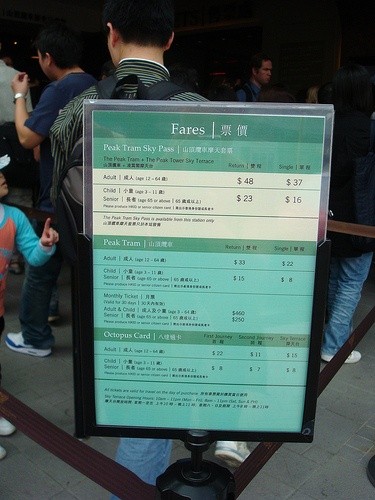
49, 0, 252, 500
202, 53, 333, 104
5, 25, 99, 357
320, 63, 375, 364
0, 153, 59, 459
0, 41, 41, 189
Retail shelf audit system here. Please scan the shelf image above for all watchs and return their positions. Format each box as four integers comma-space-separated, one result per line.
13, 93, 27, 104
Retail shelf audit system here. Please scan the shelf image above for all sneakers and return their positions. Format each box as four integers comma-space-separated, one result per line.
322, 350, 362, 363
3, 331, 51, 357
215, 441, 251, 468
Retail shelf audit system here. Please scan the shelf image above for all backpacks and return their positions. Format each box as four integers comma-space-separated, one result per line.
52, 75, 190, 264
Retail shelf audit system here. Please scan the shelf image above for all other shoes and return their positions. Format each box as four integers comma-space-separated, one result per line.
0, 446, 7, 461
48, 313, 60, 323
0, 415, 15, 436
8, 261, 21, 275
